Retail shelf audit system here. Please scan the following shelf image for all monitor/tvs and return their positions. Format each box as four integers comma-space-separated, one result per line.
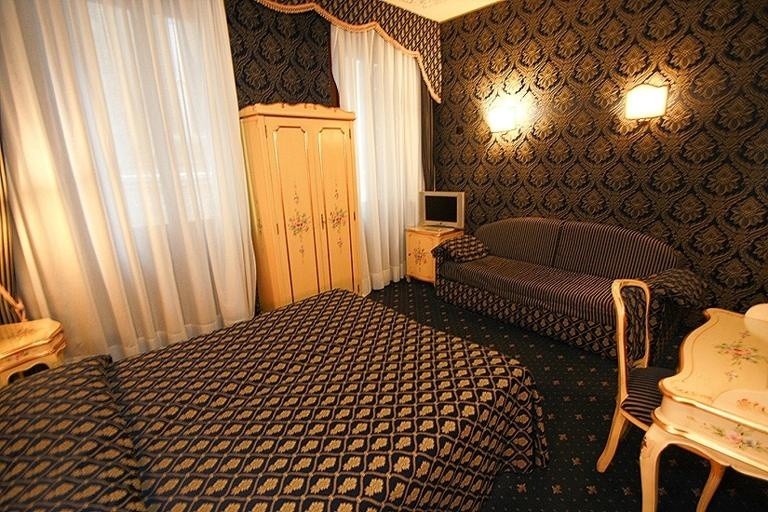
418, 191, 465, 229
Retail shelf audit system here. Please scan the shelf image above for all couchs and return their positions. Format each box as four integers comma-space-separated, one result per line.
430, 216, 709, 373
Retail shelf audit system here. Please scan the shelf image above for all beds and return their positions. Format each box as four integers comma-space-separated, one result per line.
0, 287, 552, 512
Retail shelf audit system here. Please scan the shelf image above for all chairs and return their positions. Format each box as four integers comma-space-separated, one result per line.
596, 279, 727, 512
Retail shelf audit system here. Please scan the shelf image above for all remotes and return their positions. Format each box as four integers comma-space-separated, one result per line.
424, 226, 440, 230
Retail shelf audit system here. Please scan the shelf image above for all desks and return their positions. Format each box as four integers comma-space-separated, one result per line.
0, 318, 67, 388
638, 307, 768, 511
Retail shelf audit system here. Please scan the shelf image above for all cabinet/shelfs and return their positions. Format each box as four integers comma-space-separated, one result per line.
240, 101, 366, 314
404, 225, 465, 289
